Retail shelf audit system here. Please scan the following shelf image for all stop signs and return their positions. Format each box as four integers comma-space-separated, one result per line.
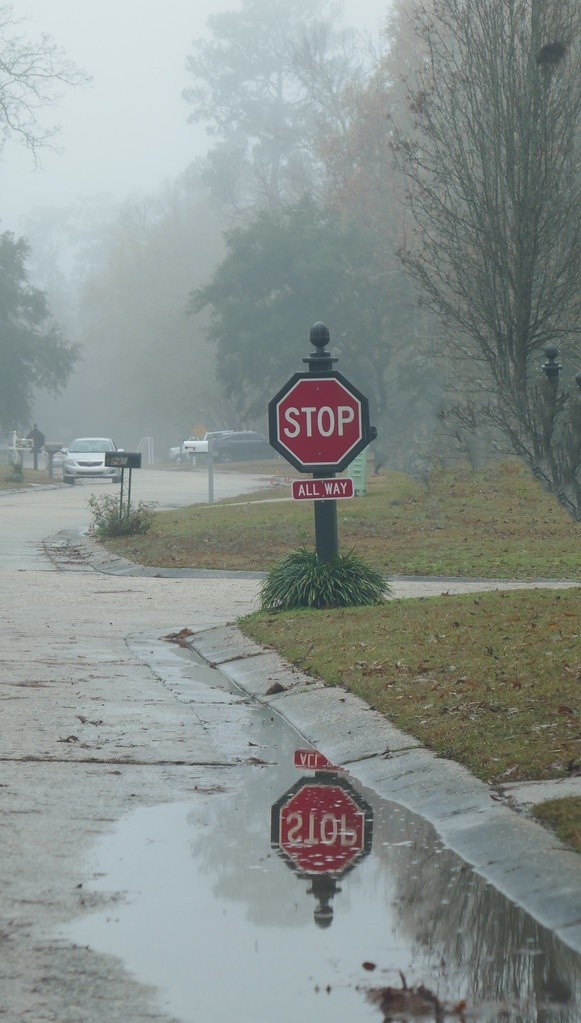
269, 775, 375, 879
268, 371, 372, 473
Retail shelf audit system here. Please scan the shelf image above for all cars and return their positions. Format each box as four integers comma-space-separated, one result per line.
207, 430, 276, 464
60, 437, 126, 484
